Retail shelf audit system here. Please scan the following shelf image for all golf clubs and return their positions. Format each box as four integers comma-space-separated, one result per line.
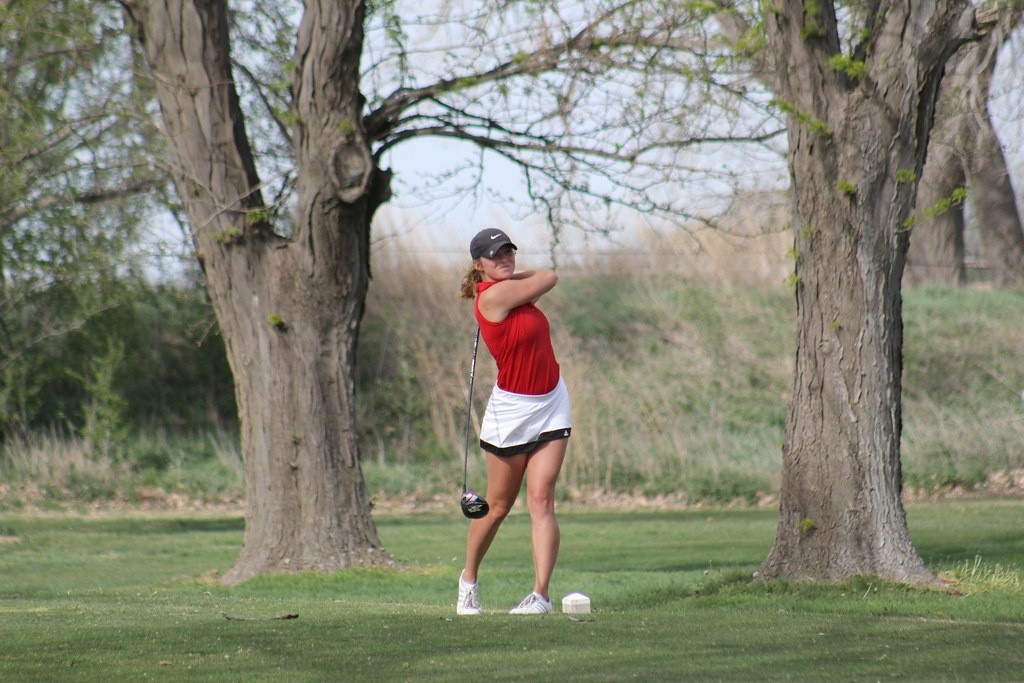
460, 325, 490, 519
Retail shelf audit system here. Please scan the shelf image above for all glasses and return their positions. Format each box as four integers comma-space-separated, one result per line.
493, 246, 516, 258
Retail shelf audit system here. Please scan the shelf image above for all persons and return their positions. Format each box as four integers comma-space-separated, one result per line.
457, 228, 570, 614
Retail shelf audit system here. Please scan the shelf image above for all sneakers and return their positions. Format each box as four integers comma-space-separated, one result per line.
509, 592, 553, 614
457, 569, 481, 615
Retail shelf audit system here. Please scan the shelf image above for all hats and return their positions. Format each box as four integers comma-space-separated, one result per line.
470, 228, 517, 259
